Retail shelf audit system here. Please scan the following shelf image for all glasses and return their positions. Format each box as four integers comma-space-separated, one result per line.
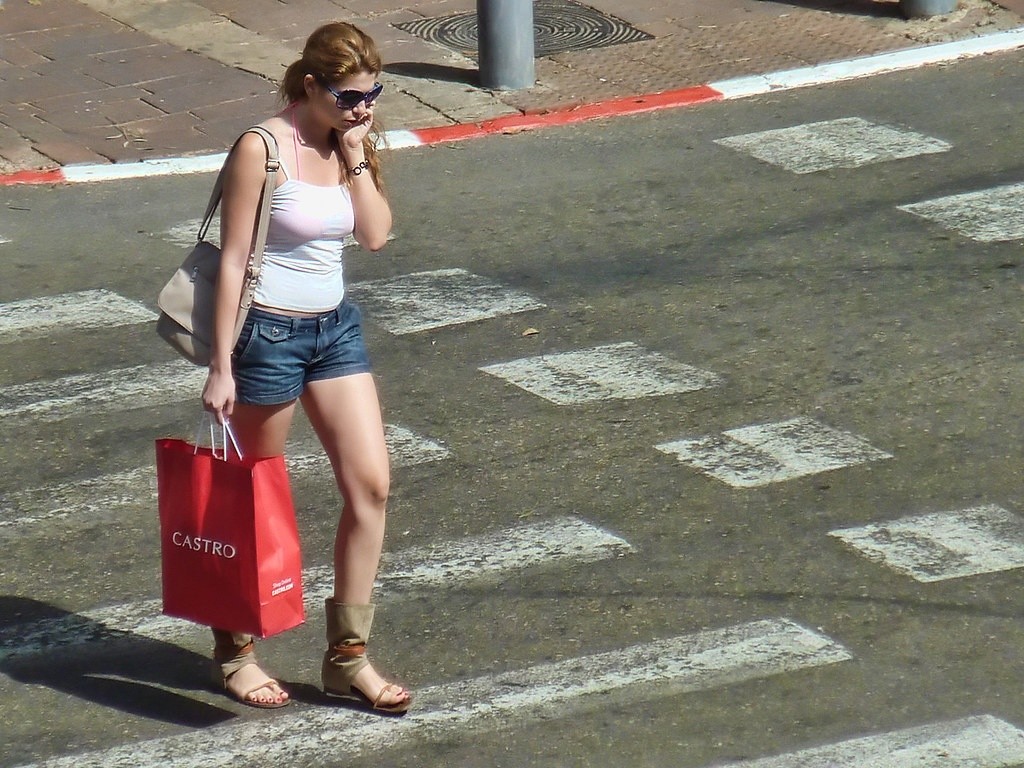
321, 80, 383, 111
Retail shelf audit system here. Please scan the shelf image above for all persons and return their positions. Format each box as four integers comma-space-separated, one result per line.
199, 23, 415, 716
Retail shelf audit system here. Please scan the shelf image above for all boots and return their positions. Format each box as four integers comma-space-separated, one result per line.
207, 627, 292, 709
320, 598, 412, 714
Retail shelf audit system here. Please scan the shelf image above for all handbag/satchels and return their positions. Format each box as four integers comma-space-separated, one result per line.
156, 413, 307, 641
155, 129, 279, 368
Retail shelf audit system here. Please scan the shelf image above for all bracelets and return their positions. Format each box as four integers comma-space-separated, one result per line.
348, 158, 370, 177
